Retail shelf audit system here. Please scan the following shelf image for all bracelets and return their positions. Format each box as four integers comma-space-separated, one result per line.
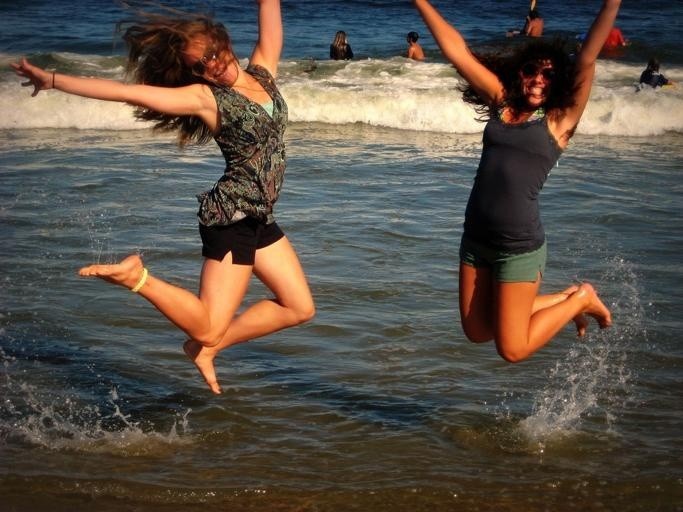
51, 71, 56, 89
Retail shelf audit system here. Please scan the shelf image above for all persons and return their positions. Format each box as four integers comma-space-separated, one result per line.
406, 32, 425, 60
639, 56, 672, 91
600, 26, 630, 63
10, 0, 318, 403
411, 1, 620, 368
525, 10, 544, 39
329, 31, 354, 60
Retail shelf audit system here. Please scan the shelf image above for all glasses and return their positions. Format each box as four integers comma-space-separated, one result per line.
522, 64, 554, 82
190, 41, 221, 77
